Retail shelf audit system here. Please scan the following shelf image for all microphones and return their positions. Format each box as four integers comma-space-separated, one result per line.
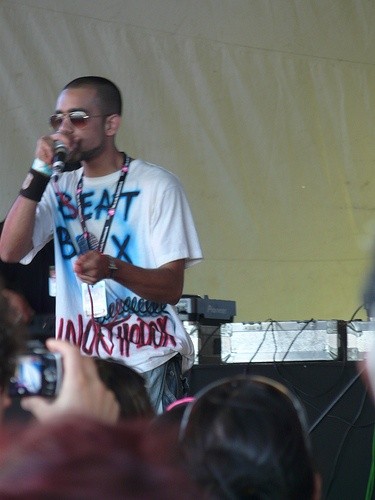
53, 140, 68, 182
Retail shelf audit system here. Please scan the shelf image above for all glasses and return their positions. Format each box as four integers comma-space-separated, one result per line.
49, 111, 106, 129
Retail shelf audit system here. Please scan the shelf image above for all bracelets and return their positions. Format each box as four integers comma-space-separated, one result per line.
107, 257, 119, 278
19, 168, 51, 202
31, 157, 53, 176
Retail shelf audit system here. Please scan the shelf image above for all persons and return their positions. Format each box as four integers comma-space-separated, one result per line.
0, 76, 203, 414
0, 267, 322, 500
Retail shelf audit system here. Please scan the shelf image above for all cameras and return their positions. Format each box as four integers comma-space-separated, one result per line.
6, 352, 62, 400
